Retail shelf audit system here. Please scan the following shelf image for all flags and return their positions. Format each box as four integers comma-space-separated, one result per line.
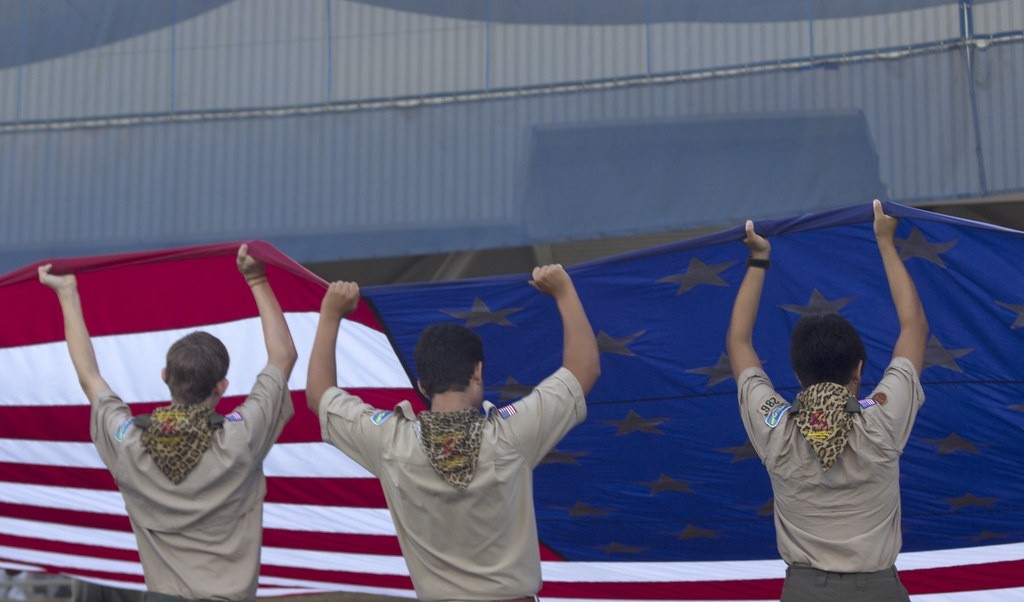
0, 201, 1024, 602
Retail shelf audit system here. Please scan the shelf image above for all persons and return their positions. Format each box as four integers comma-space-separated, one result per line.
39, 244, 299, 602
307, 264, 600, 602
726, 200, 929, 602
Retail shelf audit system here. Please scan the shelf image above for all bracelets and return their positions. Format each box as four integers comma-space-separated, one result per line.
748, 258, 770, 270
245, 274, 267, 288
